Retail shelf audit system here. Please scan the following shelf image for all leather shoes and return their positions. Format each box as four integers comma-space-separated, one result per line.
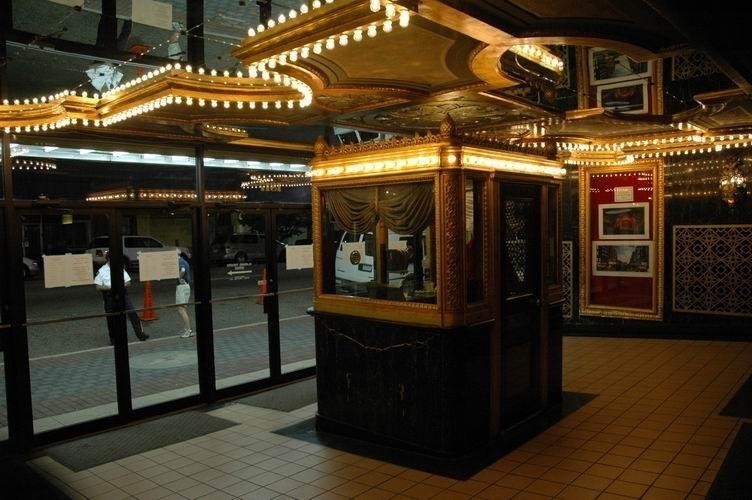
140, 334, 151, 342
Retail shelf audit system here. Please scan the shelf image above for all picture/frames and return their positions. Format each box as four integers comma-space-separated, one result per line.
597, 202, 650, 239
575, 45, 665, 118
596, 78, 648, 115
579, 158, 665, 322
589, 47, 654, 85
592, 241, 654, 278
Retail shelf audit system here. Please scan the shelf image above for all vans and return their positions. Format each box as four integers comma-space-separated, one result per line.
210, 234, 286, 264
336, 226, 428, 302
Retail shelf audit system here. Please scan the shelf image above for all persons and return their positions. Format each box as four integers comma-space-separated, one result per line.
174, 248, 194, 338
96, 250, 150, 345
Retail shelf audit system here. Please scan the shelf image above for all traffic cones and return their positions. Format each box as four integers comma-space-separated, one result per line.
138, 274, 159, 322
256, 269, 268, 305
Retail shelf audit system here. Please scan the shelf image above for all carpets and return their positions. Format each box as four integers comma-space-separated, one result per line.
233, 378, 318, 413
719, 374, 752, 420
41, 409, 240, 472
705, 422, 752, 500
271, 389, 599, 481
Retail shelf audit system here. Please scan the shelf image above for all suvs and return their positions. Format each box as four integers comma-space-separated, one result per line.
85, 235, 192, 269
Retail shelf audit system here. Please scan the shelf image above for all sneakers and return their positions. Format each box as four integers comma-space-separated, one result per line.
177, 328, 195, 339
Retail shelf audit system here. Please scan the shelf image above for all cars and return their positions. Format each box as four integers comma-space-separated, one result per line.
22, 257, 40, 280
